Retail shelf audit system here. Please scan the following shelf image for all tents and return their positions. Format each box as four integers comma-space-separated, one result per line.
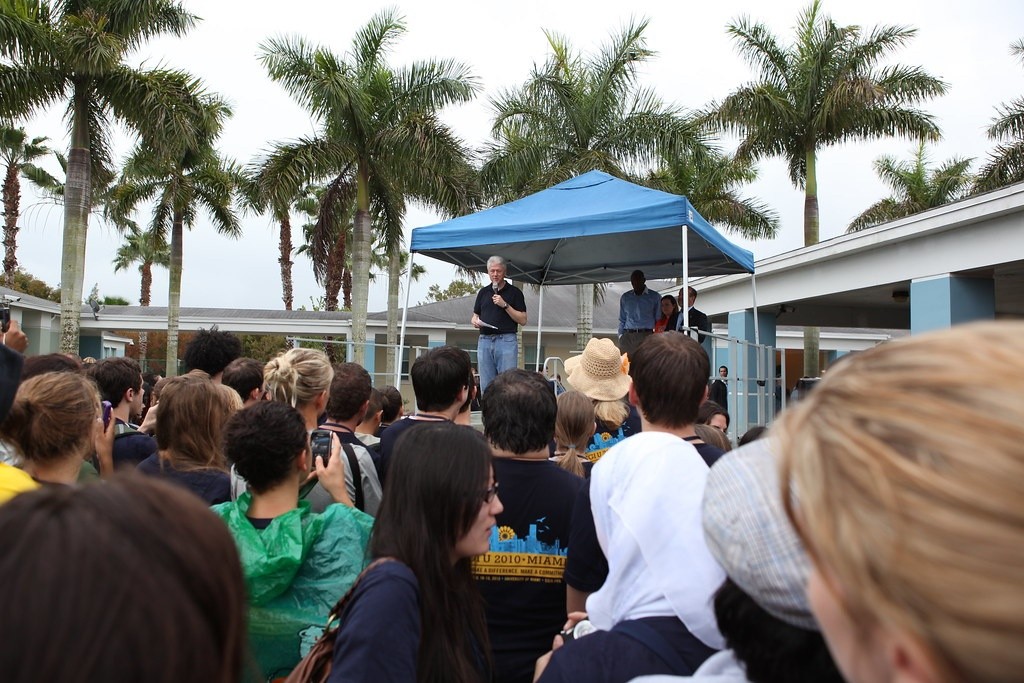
397, 169, 759, 395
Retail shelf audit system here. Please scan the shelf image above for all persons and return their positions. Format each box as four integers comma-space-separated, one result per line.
654, 295, 679, 334
471, 256, 527, 393
564, 330, 727, 614
718, 366, 729, 386
330, 421, 503, 683
378, 345, 471, 489
695, 400, 769, 452
0, 330, 404, 515
208, 400, 375, 683
616, 270, 663, 360
777, 318, 1024, 683
469, 367, 586, 683
664, 286, 707, 344
548, 337, 641, 480
628, 438, 853, 683
0, 475, 247, 683
533, 430, 727, 683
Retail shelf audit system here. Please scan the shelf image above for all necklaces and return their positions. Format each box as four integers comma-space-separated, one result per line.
416, 414, 447, 420
555, 453, 588, 460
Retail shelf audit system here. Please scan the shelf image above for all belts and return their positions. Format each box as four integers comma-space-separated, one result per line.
624, 328, 653, 334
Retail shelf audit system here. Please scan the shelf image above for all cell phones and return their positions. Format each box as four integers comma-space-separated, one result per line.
310, 429, 333, 470
101, 401, 111, 424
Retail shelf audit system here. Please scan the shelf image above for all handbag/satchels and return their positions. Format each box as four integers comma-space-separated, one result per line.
280, 559, 426, 683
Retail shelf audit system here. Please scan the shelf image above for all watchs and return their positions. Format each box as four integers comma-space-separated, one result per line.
503, 303, 509, 309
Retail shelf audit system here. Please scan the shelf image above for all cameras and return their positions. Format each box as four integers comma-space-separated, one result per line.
0, 308, 11, 332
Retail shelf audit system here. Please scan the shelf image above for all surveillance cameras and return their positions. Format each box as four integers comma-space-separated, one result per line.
5, 295, 22, 302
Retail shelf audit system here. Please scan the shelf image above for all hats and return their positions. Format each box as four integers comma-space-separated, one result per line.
700, 436, 823, 633
564, 337, 632, 401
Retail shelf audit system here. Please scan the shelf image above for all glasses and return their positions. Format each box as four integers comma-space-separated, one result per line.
463, 482, 499, 505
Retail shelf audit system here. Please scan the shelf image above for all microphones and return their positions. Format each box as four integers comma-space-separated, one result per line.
493, 282, 500, 311
